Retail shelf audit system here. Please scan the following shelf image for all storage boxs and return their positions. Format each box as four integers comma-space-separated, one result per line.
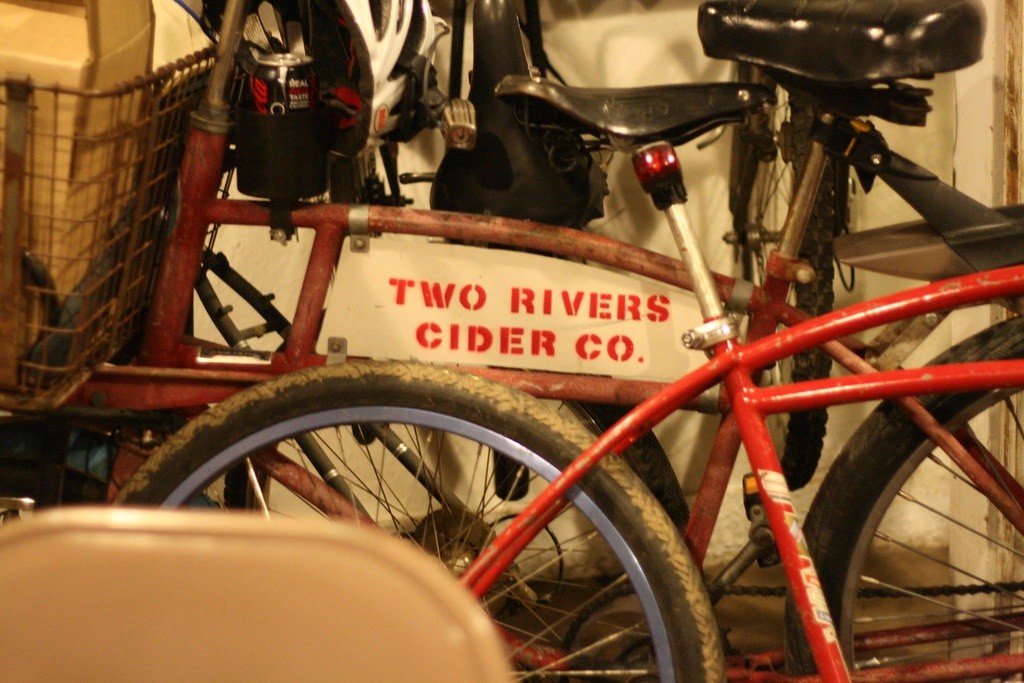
0, 0, 155, 303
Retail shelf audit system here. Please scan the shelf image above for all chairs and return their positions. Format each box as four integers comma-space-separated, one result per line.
0, 506, 515, 683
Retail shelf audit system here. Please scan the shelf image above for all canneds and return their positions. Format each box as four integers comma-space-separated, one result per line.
247, 53, 315, 115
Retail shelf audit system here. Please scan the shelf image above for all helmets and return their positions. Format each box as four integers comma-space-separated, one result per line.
205, 0, 449, 157
